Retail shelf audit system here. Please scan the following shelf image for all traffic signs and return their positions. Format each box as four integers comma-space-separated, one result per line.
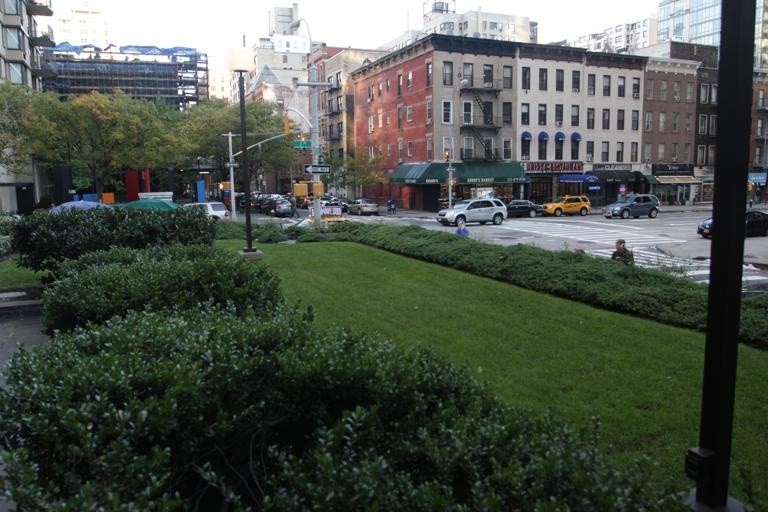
445, 166, 455, 172
304, 164, 333, 174
291, 139, 314, 148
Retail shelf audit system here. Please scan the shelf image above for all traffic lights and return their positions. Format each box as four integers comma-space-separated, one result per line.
450, 179, 456, 185
443, 147, 450, 162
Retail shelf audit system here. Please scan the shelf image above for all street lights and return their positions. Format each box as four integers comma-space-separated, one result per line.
287, 17, 323, 226
232, 68, 258, 252
226, 130, 302, 220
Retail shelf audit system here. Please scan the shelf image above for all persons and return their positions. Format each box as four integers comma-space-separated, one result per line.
455, 217, 468, 238
610, 237, 632, 267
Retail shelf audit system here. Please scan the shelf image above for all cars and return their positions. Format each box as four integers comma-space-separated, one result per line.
286, 214, 351, 230
209, 185, 377, 217
504, 199, 544, 218
696, 209, 767, 240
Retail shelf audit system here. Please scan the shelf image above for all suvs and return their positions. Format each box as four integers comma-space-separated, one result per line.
433, 197, 508, 226
542, 195, 593, 216
601, 193, 661, 219
179, 201, 227, 224
